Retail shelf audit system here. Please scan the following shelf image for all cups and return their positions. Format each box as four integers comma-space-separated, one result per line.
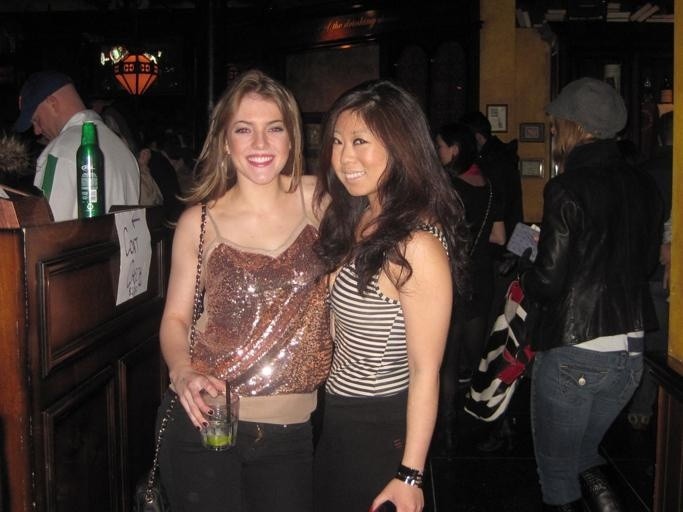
202, 392, 239, 448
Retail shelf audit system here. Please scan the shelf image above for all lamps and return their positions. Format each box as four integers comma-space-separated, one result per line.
100, 45, 164, 96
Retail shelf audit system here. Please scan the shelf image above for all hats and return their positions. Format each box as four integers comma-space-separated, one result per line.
540, 76, 628, 139
9, 70, 74, 133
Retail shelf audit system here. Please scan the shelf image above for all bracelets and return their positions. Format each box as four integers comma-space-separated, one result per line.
395, 463, 424, 489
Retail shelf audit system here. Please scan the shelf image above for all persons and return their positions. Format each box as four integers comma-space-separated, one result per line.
309, 74, 465, 511
13, 65, 142, 226
95, 98, 205, 222
432, 124, 508, 462
148, 68, 347, 510
460, 111, 525, 231
499, 77, 664, 512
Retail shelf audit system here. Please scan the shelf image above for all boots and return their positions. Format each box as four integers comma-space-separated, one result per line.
578, 459, 631, 512
541, 497, 585, 512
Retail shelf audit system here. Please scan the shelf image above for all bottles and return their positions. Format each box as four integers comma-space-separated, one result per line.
75, 122, 107, 219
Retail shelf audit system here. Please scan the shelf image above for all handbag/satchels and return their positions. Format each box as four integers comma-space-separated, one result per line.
131, 467, 172, 512
451, 255, 477, 302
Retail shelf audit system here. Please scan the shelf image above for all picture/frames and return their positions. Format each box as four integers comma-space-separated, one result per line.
517, 120, 544, 145
483, 101, 509, 134
517, 157, 543, 180
